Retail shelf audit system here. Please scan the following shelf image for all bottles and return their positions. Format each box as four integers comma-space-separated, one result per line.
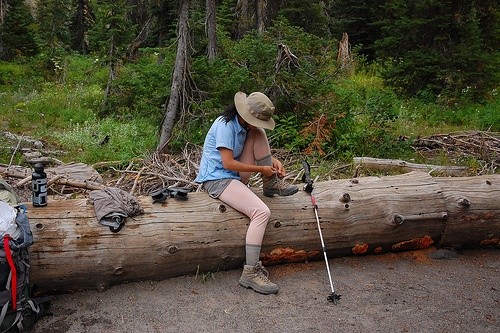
32, 163, 48, 207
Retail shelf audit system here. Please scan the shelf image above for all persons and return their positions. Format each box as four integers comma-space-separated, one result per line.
197, 90, 300, 293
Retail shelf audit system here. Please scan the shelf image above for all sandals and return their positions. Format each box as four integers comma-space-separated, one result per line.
152, 188, 170, 204
168, 188, 191, 200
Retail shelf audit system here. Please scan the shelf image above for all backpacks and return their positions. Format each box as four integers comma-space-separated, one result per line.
0, 243, 53, 333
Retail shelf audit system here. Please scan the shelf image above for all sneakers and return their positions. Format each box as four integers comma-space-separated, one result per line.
261, 171, 299, 196
239, 261, 279, 294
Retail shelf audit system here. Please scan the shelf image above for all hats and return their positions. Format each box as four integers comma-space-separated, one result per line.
234, 91, 276, 130
